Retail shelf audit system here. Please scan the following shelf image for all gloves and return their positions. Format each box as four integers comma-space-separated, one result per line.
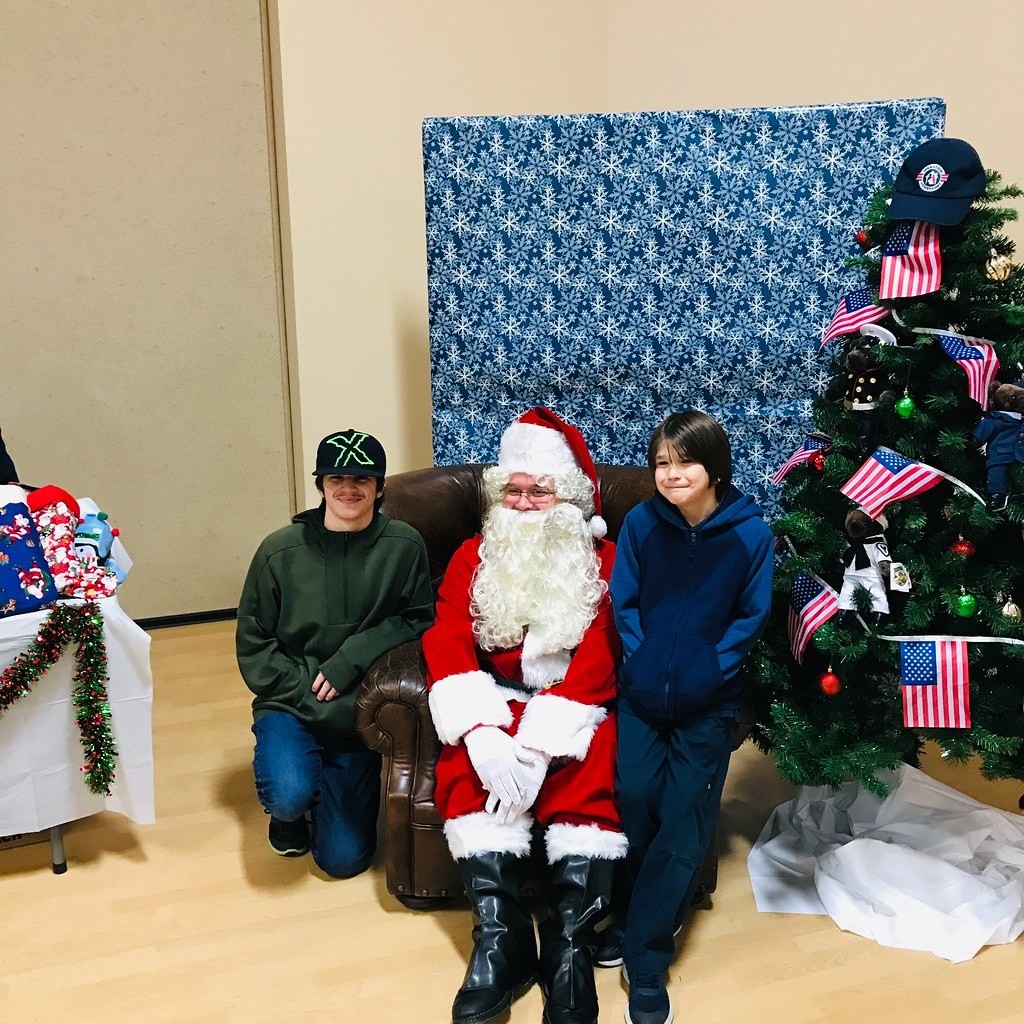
464, 726, 534, 806
482, 735, 553, 826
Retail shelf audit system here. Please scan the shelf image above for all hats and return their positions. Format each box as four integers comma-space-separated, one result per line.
499, 406, 607, 541
312, 429, 386, 478
887, 137, 985, 227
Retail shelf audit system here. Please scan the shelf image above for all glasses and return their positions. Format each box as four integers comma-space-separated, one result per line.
500, 487, 556, 503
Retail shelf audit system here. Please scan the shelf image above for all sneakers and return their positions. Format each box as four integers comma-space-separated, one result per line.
264, 808, 310, 856
623, 962, 673, 1024
594, 923, 683, 967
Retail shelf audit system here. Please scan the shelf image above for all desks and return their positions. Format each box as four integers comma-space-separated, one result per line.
0, 595, 156, 874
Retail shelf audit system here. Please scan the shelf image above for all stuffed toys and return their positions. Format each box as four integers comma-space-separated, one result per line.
836, 505, 891, 615
973, 382, 1024, 512
843, 325, 896, 411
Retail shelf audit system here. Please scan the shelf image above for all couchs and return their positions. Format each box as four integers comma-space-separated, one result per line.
359, 462, 653, 912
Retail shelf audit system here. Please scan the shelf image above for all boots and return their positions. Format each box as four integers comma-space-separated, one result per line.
538, 855, 610, 1024
452, 852, 537, 1024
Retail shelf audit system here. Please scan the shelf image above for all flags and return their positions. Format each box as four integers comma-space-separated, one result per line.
818, 287, 895, 353
840, 450, 946, 522
878, 222, 942, 300
774, 437, 833, 484
940, 335, 1000, 410
900, 640, 971, 728
786, 570, 839, 665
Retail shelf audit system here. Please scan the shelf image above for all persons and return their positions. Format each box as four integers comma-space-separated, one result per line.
423, 405, 628, 1024
235, 429, 433, 880
593, 412, 776, 1024
